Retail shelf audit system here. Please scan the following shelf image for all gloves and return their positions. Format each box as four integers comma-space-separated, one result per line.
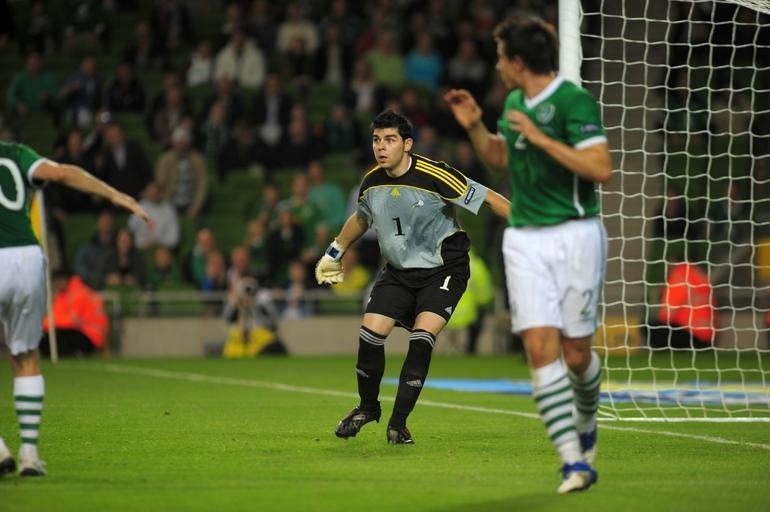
314, 240, 345, 287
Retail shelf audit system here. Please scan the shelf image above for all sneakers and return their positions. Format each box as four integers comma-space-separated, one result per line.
18, 460, 47, 475
386, 423, 413, 443
0, 449, 15, 476
335, 401, 381, 440
557, 423, 598, 496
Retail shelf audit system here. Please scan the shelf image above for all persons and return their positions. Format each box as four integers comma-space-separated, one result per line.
39, 261, 110, 358
314, 110, 512, 445
641, 242, 719, 353
1, 2, 557, 356
222, 276, 287, 356
444, 18, 612, 495
0, 140, 150, 476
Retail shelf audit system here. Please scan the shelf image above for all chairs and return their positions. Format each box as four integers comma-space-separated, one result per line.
15, 68, 494, 314
